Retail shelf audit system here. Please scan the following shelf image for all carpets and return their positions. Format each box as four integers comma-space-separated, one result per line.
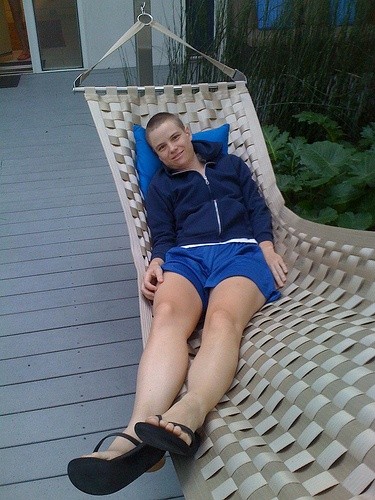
0, 74, 21, 87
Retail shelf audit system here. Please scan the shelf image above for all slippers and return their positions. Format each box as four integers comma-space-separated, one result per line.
134, 415, 200, 459
67, 433, 167, 496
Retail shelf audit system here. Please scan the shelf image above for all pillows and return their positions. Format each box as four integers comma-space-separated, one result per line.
132, 123, 229, 199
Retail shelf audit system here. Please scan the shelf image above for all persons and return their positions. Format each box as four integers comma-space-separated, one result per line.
67, 112, 289, 496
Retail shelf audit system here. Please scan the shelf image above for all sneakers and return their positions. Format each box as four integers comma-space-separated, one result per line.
17, 50, 30, 60
